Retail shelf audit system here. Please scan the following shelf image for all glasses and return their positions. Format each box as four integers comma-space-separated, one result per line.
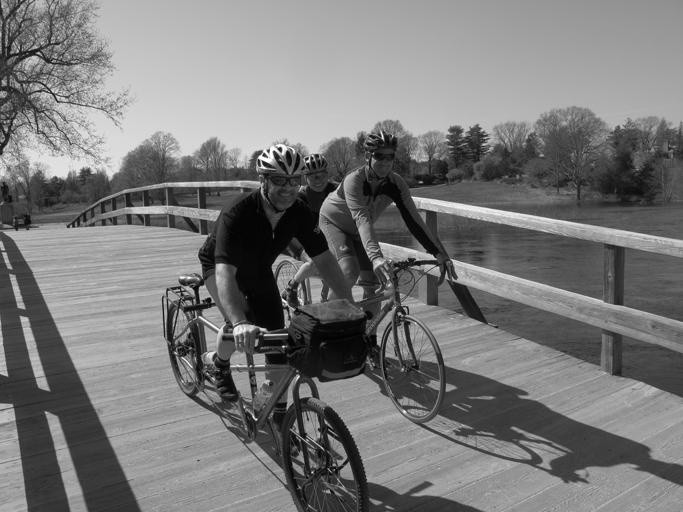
370, 151, 395, 161
263, 175, 301, 187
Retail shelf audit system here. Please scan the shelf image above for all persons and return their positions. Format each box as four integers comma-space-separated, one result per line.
280, 152, 340, 309
317, 130, 459, 371
198, 143, 354, 402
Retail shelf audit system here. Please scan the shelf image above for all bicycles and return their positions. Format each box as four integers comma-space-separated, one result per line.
158, 270, 372, 511
272, 248, 458, 427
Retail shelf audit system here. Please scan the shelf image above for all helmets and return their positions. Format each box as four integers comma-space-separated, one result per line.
363, 130, 397, 153
302, 154, 328, 174
255, 144, 305, 175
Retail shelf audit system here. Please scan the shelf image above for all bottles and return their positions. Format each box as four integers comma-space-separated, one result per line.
252, 377, 274, 413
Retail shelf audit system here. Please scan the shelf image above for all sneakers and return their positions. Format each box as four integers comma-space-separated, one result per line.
205, 351, 239, 402
368, 345, 381, 370
280, 286, 301, 309
271, 413, 300, 457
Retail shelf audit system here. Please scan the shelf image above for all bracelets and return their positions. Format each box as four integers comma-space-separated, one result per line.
231, 319, 249, 328
